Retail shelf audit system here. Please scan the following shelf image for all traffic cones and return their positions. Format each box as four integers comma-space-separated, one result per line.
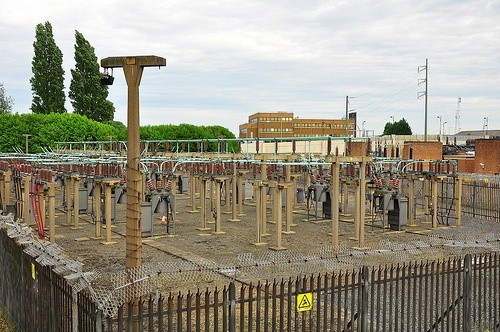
160, 213, 166, 225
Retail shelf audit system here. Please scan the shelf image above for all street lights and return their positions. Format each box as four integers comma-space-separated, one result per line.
391, 116, 395, 124
437, 116, 441, 135
443, 121, 447, 134
